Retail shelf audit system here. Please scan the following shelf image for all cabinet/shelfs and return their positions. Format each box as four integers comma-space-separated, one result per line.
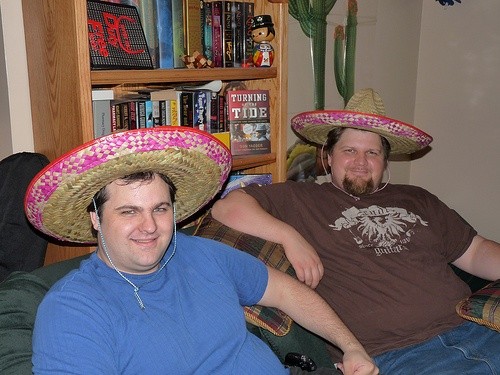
25, 0, 286, 266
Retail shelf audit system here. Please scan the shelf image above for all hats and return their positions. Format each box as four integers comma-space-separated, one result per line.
24, 126, 233, 244
291, 88, 433, 156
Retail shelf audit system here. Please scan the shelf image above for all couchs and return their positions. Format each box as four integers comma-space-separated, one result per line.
0, 252, 491, 375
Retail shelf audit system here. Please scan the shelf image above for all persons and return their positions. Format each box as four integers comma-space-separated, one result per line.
211, 88, 500, 375
24, 126, 379, 375
250, 15, 275, 68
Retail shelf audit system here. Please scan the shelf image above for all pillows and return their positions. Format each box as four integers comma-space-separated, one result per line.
188, 208, 301, 336
456, 278, 500, 333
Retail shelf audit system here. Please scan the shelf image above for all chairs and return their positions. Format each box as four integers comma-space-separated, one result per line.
0, 152, 49, 284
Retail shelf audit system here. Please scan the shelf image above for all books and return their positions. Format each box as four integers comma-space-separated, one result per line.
87, 0, 254, 71
92, 80, 271, 155
221, 171, 272, 199
